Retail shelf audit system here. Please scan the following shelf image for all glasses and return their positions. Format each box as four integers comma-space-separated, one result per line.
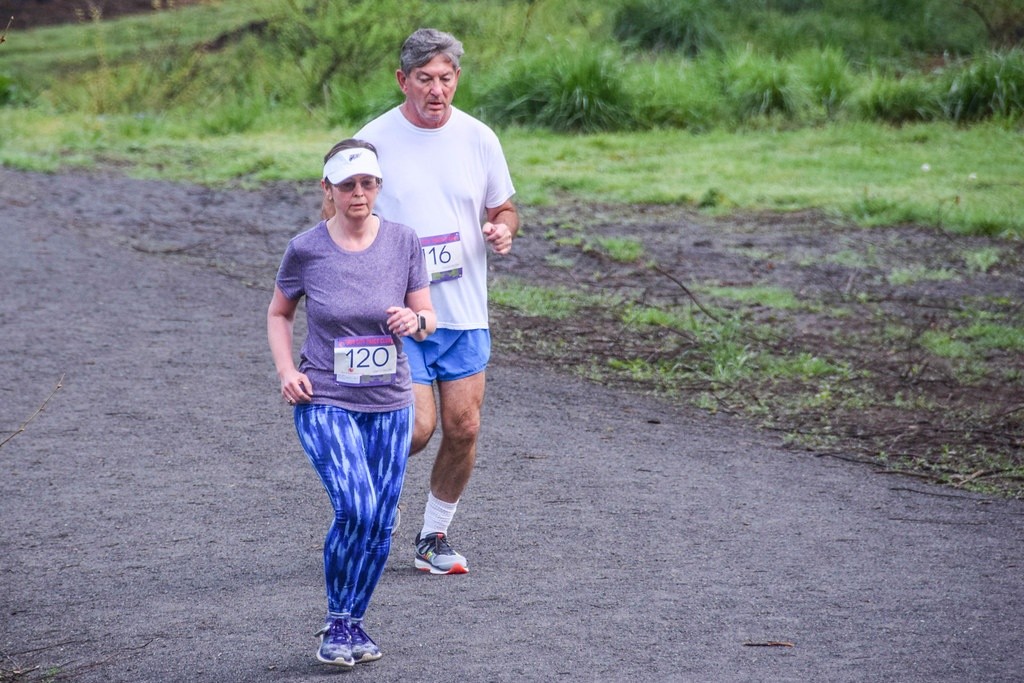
324, 175, 380, 193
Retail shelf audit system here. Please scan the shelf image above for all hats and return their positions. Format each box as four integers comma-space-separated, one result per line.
322, 147, 382, 185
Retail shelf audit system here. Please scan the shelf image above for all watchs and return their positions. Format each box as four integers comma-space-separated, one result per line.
414, 311, 427, 333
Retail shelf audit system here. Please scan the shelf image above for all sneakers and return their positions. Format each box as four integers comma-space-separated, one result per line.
391, 506, 470, 575
314, 618, 382, 666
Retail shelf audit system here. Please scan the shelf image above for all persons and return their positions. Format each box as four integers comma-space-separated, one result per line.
265, 139, 437, 667
319, 26, 519, 576
